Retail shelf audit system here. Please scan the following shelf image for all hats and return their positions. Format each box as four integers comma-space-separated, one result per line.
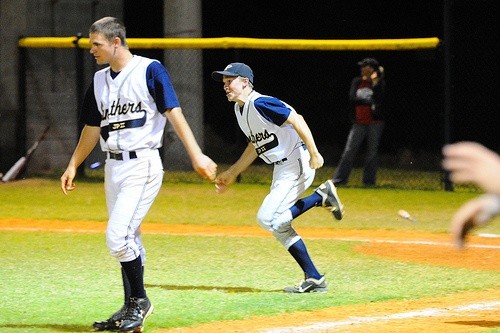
358, 58, 378, 66
211, 62, 253, 84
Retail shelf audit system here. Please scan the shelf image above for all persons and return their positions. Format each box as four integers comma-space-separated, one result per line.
442, 139, 500, 245
331, 58, 385, 187
61, 16, 217, 333
214, 63, 345, 293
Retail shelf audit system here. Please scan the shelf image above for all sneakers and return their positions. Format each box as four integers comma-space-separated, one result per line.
284, 273, 327, 294
315, 179, 344, 221
109, 303, 130, 332
121, 297, 153, 331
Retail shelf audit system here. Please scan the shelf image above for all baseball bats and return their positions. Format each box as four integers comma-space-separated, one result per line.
398, 209, 416, 222
2, 127, 48, 182
